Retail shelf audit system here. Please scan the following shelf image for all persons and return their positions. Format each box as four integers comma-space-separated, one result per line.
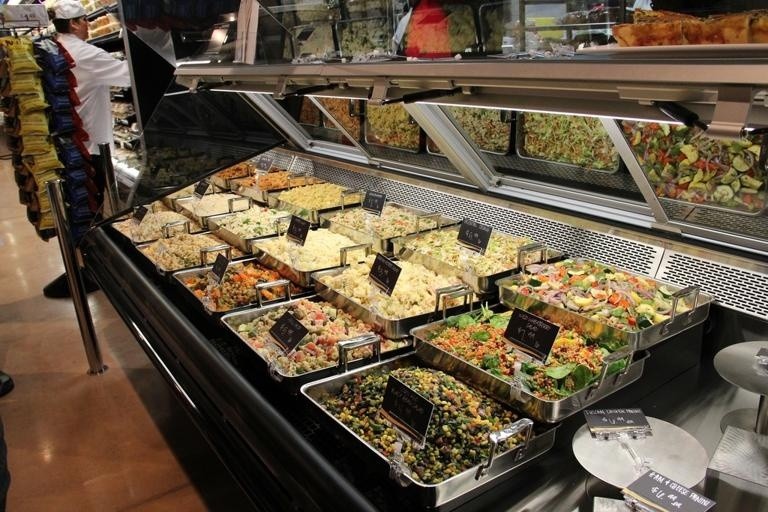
47, 1, 133, 219
1, 373, 15, 400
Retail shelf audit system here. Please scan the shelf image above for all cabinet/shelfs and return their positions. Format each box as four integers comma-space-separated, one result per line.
51, 2, 768, 511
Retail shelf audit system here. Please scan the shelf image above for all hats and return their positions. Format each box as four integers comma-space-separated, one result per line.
47, 0, 87, 21
